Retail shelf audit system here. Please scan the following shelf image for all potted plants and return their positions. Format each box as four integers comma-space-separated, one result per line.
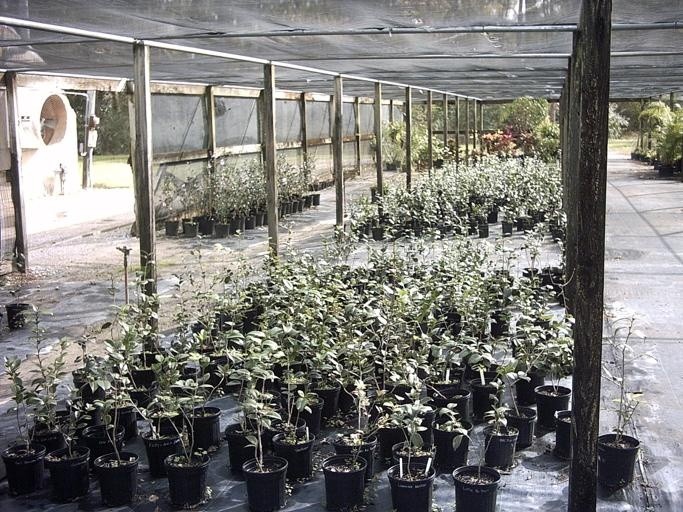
49, 444, 91, 501
321, 440, 369, 510
436, 405, 472, 467
244, 417, 289, 512
46, 238, 576, 439
89, 422, 124, 459
332, 391, 383, 479
552, 407, 572, 455
599, 318, 649, 491
162, 149, 321, 235
187, 363, 227, 451
144, 360, 183, 477
451, 439, 502, 510
487, 424, 518, 470
96, 387, 140, 508
3, 356, 44, 497
166, 397, 212, 509
389, 410, 434, 463
506, 408, 536, 445
273, 429, 316, 478
225, 390, 265, 479
349, 185, 563, 238
388, 427, 434, 509
630, 105, 683, 177
5, 251, 32, 330
